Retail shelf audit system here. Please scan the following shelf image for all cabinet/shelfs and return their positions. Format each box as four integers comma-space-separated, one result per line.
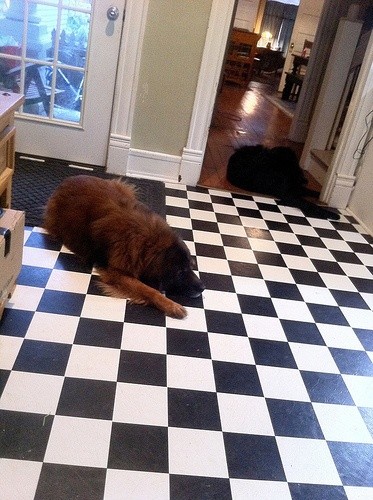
224, 30, 262, 88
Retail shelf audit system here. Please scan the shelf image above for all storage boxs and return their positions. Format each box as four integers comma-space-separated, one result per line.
0, 207, 26, 319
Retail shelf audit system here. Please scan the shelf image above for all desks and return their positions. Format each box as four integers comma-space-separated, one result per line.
282, 54, 310, 101
0, 89, 25, 208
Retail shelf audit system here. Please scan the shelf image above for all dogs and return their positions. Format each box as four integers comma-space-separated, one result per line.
40, 172, 206, 320
225, 143, 342, 221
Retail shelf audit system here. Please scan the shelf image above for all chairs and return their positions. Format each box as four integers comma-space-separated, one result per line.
285, 39, 313, 102
0, 56, 65, 119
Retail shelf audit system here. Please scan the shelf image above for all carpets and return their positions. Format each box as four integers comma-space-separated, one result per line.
12, 151, 166, 228
261, 92, 297, 118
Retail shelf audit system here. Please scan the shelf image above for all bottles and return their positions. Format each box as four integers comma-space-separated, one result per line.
301, 49, 306, 58
